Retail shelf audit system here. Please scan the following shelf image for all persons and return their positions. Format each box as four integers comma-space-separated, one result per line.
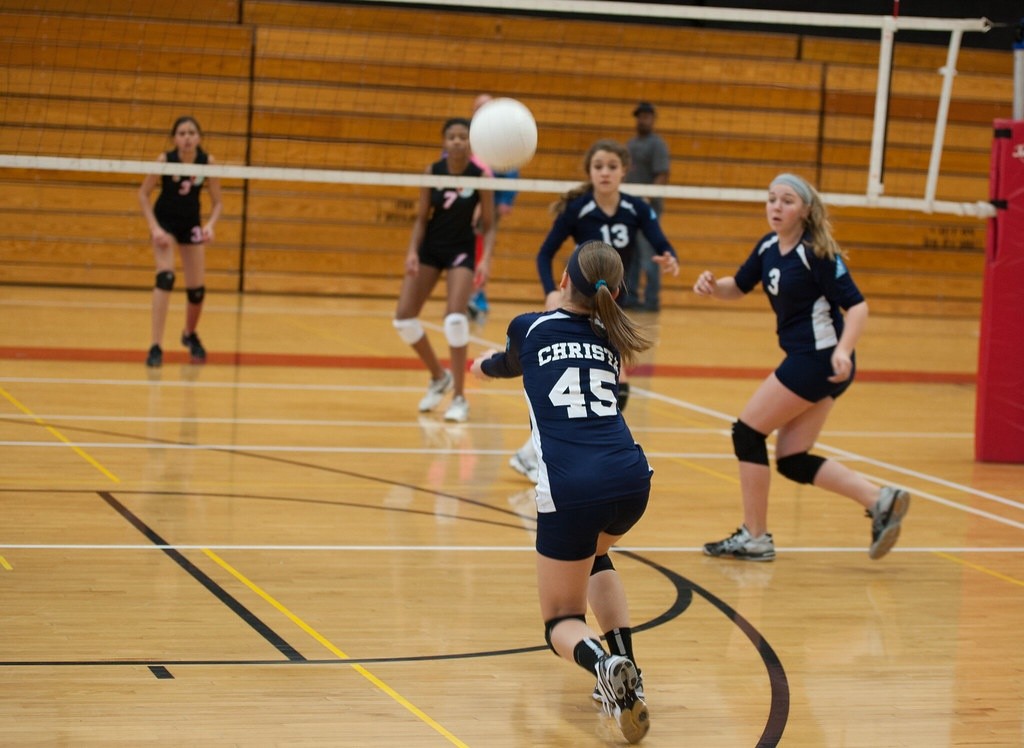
623, 102, 669, 311
140, 115, 223, 368
443, 94, 518, 320
470, 239, 657, 744
695, 173, 910, 563
392, 117, 495, 422
509, 141, 681, 483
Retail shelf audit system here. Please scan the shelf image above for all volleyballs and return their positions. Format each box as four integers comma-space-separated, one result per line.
469, 95, 538, 173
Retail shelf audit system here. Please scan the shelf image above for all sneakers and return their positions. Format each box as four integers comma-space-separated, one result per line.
508, 452, 538, 483
418, 369, 454, 412
703, 524, 775, 561
594, 655, 651, 743
181, 331, 205, 358
146, 344, 162, 367
445, 396, 469, 421
864, 486, 910, 560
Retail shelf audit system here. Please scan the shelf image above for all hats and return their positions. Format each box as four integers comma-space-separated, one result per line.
632, 102, 654, 116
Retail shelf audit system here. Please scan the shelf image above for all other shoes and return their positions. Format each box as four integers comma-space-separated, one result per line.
621, 301, 658, 312
